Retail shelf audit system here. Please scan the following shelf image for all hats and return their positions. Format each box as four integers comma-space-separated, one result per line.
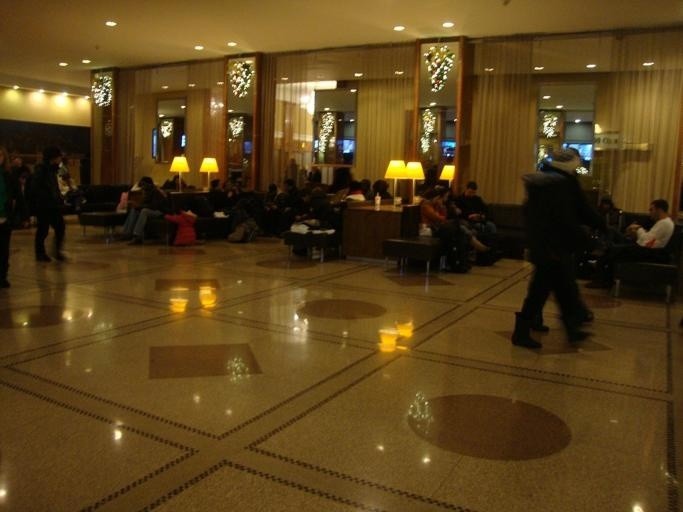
42, 144, 62, 160
546, 150, 583, 170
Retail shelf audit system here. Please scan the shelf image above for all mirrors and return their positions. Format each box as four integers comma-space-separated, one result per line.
156, 99, 186, 162
311, 88, 357, 166
535, 84, 595, 174
226, 56, 256, 188
416, 40, 459, 187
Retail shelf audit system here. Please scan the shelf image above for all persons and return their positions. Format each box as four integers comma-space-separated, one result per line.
418, 180, 488, 271
512, 144, 674, 349
265, 174, 393, 259
119, 175, 277, 244
0, 144, 83, 288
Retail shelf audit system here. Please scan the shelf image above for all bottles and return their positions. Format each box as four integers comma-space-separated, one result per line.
374, 192, 382, 211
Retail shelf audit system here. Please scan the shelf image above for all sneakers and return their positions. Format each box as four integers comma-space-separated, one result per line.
117, 233, 143, 245
35, 251, 71, 262
511, 316, 594, 350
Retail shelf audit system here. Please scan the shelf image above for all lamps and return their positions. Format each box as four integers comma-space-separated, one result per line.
439, 164, 456, 187
406, 161, 426, 201
169, 156, 191, 192
198, 157, 219, 189
384, 159, 409, 201
